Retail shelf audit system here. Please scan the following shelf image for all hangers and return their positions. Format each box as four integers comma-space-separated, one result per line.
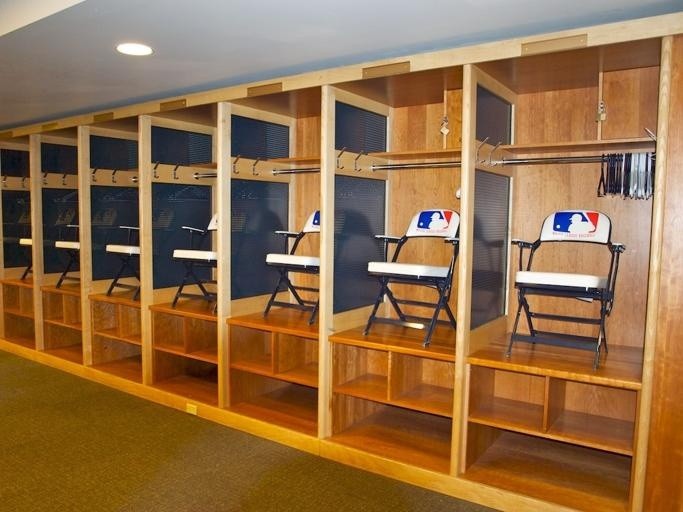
596, 152, 652, 201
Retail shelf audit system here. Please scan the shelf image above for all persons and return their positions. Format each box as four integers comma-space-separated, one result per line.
427, 212, 449, 232
567, 213, 596, 234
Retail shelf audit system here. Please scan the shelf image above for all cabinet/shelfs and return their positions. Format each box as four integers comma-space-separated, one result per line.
76, 110, 146, 402
324, 64, 468, 489
0, 124, 37, 361
34, 120, 87, 378
468, 35, 668, 512
141, 98, 224, 423
220, 87, 326, 460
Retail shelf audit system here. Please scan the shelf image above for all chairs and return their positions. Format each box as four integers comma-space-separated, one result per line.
171, 213, 217, 311
362, 208, 459, 347
264, 211, 320, 326
53, 225, 80, 291
105, 226, 139, 300
504, 210, 624, 371
17, 239, 32, 281
